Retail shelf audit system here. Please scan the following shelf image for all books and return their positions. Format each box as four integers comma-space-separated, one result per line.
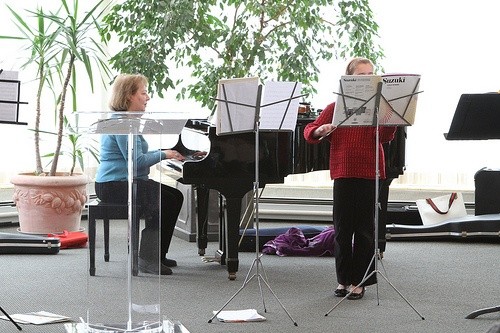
332, 74, 420, 125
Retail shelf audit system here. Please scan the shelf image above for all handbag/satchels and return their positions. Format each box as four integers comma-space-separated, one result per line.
48, 230, 88, 249
416, 191, 468, 226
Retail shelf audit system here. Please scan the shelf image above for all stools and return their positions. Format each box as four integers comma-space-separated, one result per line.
85, 198, 142, 277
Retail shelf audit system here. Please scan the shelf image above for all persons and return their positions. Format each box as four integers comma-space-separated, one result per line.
378, 124, 407, 261
94, 74, 185, 274
303, 57, 398, 300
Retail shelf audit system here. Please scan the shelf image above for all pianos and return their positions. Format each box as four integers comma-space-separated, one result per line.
155, 117, 408, 282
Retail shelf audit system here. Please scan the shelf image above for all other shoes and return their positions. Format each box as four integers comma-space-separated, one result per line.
162, 258, 177, 267
138, 257, 172, 275
335, 289, 347, 297
346, 286, 364, 300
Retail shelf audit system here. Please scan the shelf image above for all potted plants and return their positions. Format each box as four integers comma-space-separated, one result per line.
0, 0, 116, 235
